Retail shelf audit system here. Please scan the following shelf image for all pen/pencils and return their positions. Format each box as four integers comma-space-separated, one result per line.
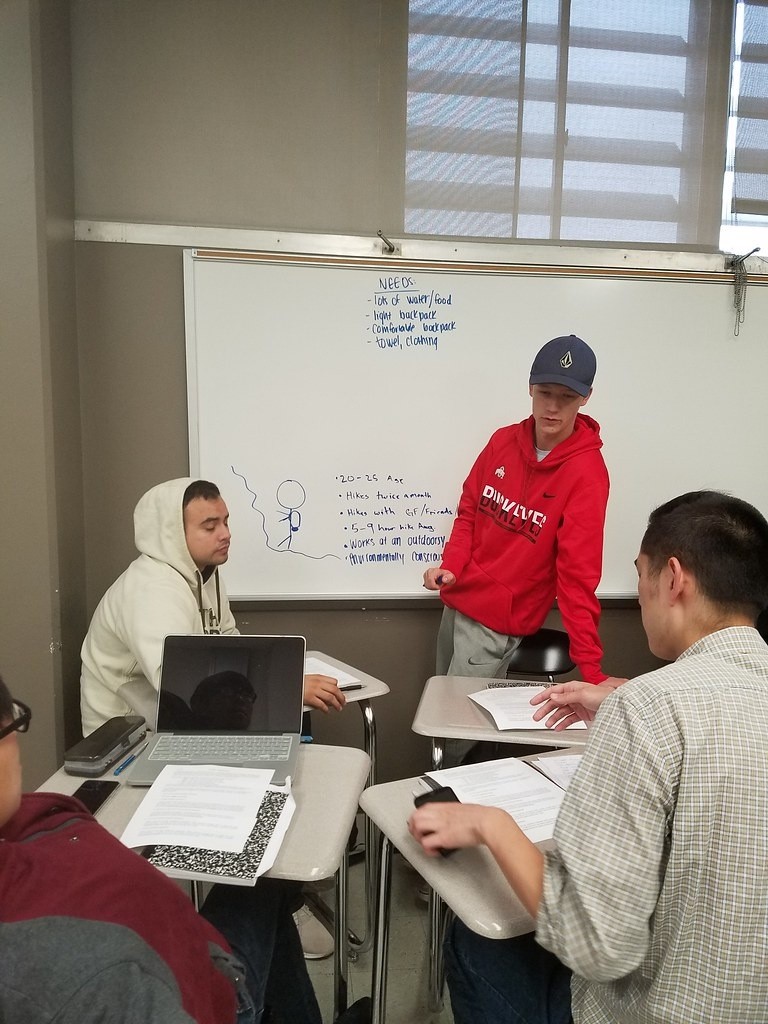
340, 685, 367, 691
112, 741, 150, 776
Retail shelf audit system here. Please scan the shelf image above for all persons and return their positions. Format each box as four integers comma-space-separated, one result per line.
0, 679, 380, 1024
79, 477, 346, 960
422, 334, 631, 908
408, 490, 768, 1024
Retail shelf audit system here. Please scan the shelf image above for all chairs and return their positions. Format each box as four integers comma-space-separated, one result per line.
460, 627, 577, 763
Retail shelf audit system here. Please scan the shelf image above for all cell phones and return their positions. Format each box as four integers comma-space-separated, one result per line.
415, 787, 462, 858
73, 780, 121, 817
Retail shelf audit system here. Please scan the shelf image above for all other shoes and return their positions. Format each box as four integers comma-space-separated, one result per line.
419, 879, 445, 903
347, 997, 372, 1024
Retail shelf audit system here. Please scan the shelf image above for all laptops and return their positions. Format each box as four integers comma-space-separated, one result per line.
127, 635, 306, 789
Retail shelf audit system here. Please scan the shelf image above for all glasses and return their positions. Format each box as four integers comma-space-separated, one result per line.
0, 700, 32, 739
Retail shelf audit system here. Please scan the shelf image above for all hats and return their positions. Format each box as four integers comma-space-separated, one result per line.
529, 335, 597, 397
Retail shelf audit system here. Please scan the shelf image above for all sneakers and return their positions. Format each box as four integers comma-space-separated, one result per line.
293, 904, 335, 960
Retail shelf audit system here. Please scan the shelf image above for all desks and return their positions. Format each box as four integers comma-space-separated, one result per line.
30, 651, 593, 1024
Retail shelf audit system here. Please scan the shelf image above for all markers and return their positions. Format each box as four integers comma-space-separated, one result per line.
421, 576, 443, 587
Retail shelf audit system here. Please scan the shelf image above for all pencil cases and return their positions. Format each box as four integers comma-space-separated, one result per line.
62, 714, 147, 778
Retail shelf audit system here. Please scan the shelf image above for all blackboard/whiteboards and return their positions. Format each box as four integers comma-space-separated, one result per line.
183, 256, 768, 601
